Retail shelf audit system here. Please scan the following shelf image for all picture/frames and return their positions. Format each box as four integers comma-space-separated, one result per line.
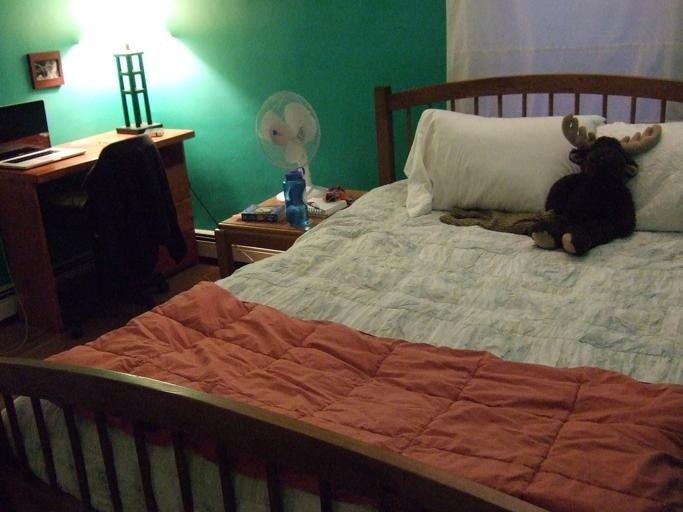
27, 50, 65, 91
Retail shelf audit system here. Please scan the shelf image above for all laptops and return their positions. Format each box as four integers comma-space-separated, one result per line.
0, 100, 86, 170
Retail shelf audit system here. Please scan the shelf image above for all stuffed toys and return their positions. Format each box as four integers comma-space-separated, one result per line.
527, 110, 662, 256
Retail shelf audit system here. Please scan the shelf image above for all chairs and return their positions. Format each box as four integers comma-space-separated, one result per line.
52, 135, 187, 341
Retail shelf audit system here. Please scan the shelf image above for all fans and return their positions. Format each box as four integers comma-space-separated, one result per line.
255, 90, 329, 203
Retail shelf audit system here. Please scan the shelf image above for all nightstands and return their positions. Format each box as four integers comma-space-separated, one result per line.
214, 190, 368, 279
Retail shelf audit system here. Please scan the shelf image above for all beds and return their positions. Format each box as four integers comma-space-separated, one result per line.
0, 74, 683, 512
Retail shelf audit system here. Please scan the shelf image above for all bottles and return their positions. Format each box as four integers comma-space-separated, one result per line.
281, 167, 308, 226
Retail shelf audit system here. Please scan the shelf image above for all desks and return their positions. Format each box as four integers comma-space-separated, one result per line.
0, 126, 199, 335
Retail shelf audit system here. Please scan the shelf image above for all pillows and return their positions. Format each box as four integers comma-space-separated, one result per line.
403, 108, 608, 219
595, 120, 682, 232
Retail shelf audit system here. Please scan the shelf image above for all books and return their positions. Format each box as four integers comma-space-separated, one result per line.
303, 197, 348, 218
239, 203, 285, 222
